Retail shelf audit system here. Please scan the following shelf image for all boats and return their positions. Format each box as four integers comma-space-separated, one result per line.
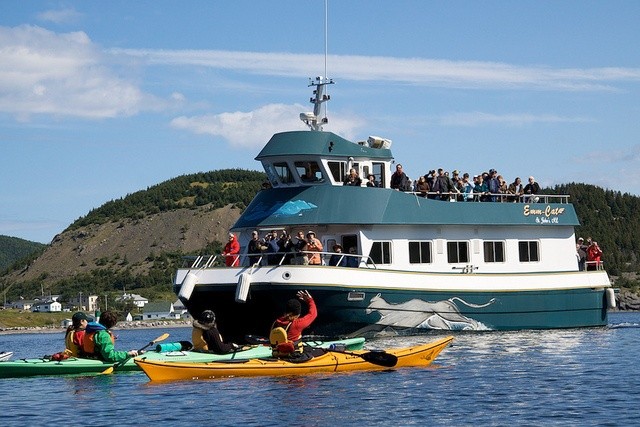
0, 351, 14, 361
0, 336, 155, 379
135, 337, 366, 363
135, 336, 454, 382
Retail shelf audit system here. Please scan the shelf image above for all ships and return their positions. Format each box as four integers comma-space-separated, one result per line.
173, 0, 617, 338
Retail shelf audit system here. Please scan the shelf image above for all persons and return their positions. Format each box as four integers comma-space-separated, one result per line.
507, 177, 524, 203
472, 174, 489, 202
444, 172, 461, 201
220, 233, 240, 267
277, 231, 295, 263
435, 168, 452, 201
247, 231, 262, 268
366, 175, 378, 187
576, 244, 586, 271
343, 169, 362, 187
497, 175, 508, 202
83, 311, 138, 364
463, 173, 476, 202
452, 169, 466, 201
423, 169, 439, 200
270, 230, 280, 265
577, 238, 583, 250
65, 312, 93, 358
586, 242, 602, 271
524, 176, 541, 203
269, 289, 323, 359
416, 176, 430, 199
294, 231, 309, 265
329, 244, 347, 266
191, 310, 239, 354
584, 237, 592, 251
481, 168, 502, 201
301, 231, 323, 265
391, 164, 406, 192
257, 234, 271, 251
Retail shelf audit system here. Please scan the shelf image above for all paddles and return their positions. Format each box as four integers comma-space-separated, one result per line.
101, 332, 170, 374
312, 346, 399, 366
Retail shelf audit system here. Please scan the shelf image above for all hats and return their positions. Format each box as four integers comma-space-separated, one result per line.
333, 244, 343, 249
307, 230, 316, 238
286, 298, 301, 316
202, 310, 215, 323
72, 312, 93, 322
